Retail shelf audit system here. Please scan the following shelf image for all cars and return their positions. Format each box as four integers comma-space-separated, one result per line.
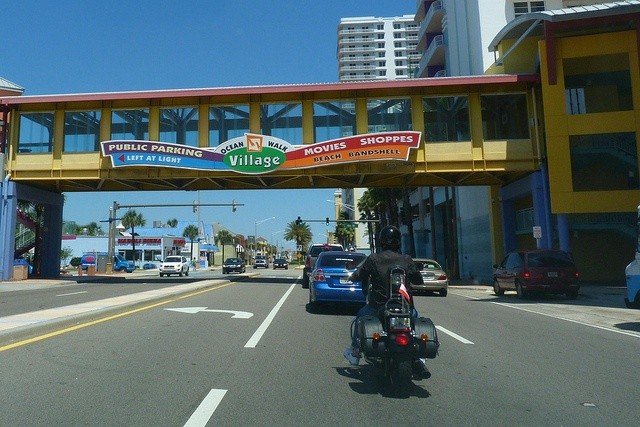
405, 257, 448, 297
273, 259, 288, 270
309, 250, 372, 305
223, 258, 246, 274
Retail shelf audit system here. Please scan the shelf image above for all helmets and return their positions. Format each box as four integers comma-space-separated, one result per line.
380, 227, 401, 248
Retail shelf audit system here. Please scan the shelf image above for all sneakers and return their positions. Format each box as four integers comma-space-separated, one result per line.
345, 351, 359, 365
416, 358, 425, 364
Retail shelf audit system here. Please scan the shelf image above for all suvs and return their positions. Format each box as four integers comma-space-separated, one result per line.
159, 256, 190, 276
493, 249, 581, 297
252, 255, 270, 269
303, 243, 345, 288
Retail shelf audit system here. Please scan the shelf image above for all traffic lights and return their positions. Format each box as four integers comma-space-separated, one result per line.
326, 217, 329, 226
296, 217, 305, 225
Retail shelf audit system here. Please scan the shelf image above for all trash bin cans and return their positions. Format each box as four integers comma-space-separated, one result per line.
12, 259, 29, 280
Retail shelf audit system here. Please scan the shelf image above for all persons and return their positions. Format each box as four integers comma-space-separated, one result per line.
344, 226, 425, 367
191, 257, 198, 272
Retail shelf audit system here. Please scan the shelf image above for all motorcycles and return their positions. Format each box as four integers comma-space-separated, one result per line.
344, 260, 439, 392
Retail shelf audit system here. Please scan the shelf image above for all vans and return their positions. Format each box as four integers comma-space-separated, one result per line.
80, 252, 135, 273
13, 257, 33, 275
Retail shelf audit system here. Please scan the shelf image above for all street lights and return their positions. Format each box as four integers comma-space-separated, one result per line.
255, 216, 276, 240
